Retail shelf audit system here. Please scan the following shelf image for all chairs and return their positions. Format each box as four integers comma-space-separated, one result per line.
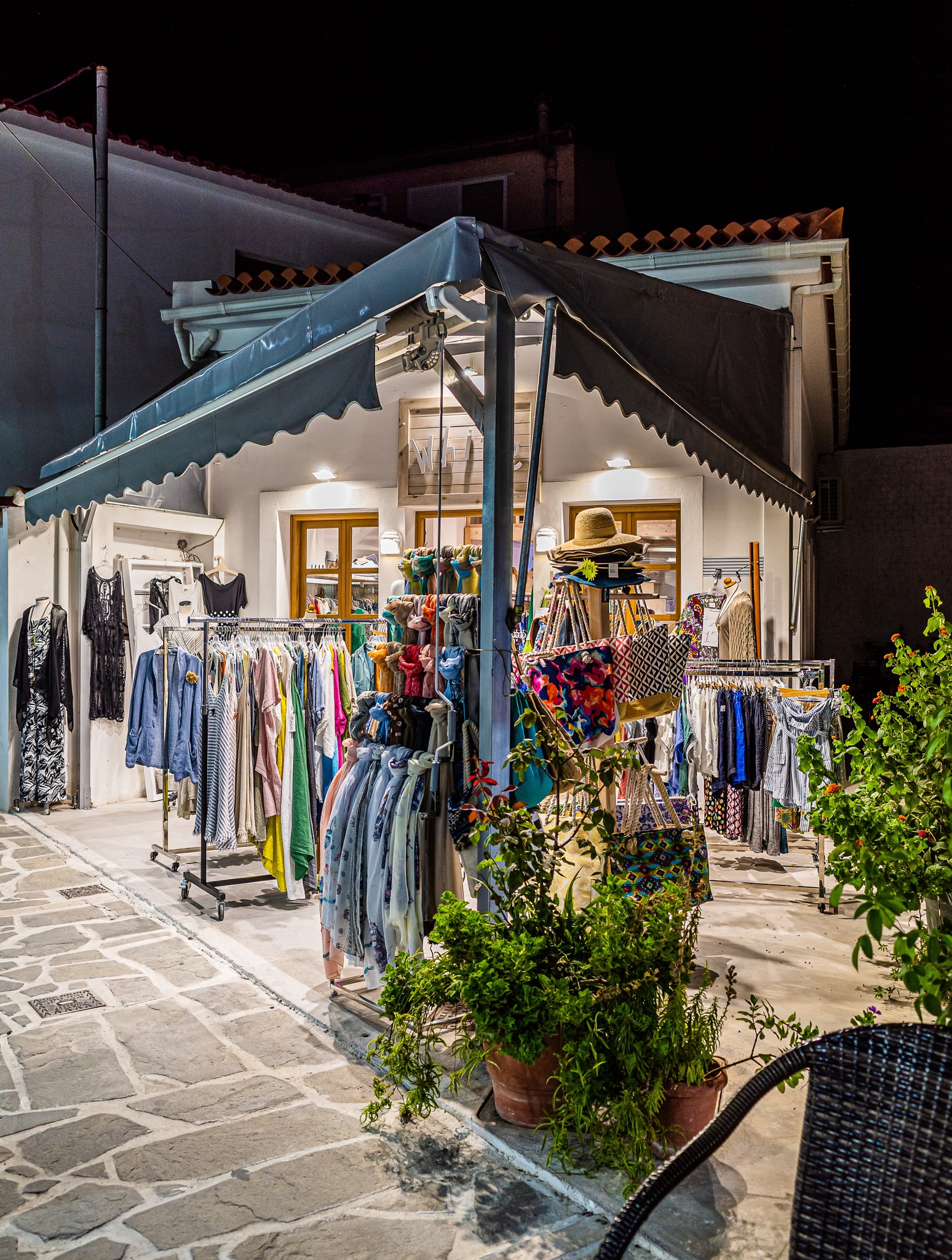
592, 1024, 952, 1260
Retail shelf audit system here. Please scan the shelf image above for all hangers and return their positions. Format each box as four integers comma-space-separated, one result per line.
90, 546, 388, 665
679, 570, 845, 714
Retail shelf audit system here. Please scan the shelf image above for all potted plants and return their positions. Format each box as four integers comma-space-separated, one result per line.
361, 706, 827, 1196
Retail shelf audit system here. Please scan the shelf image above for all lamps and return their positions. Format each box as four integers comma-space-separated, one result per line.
312, 466, 337, 480
462, 367, 478, 376
607, 458, 631, 467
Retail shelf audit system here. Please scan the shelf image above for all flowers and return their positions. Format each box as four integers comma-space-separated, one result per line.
778, 586, 952, 1030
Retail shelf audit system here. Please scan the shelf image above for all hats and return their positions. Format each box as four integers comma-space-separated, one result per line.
545, 507, 652, 589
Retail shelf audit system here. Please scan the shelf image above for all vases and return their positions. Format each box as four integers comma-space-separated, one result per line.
924, 892, 952, 940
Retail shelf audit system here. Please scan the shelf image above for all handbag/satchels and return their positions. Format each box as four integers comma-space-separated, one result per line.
510, 639, 587, 808
524, 756, 602, 916
611, 745, 714, 909
511, 577, 693, 748
